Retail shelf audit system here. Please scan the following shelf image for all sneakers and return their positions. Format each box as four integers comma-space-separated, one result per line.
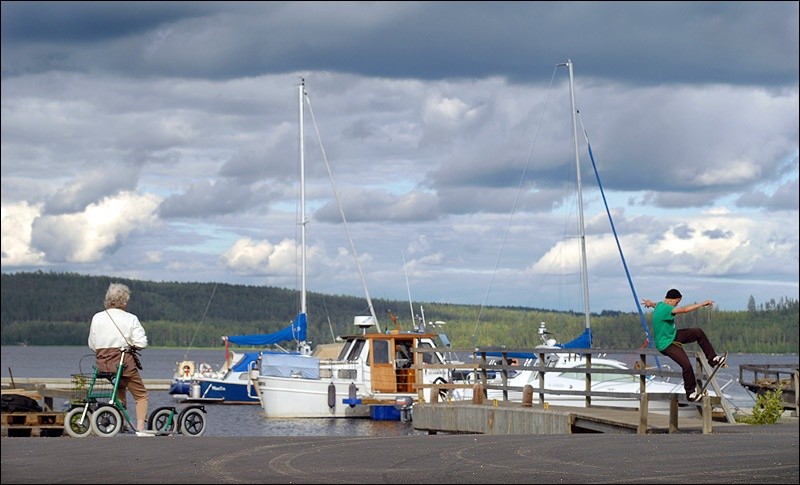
686, 392, 703, 402
708, 355, 725, 368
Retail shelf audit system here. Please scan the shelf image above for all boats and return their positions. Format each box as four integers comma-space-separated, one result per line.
251, 312, 587, 417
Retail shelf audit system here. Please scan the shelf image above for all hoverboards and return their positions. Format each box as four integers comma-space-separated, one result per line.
63, 344, 207, 438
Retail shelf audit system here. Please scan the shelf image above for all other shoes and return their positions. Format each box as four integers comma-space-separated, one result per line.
135, 432, 155, 437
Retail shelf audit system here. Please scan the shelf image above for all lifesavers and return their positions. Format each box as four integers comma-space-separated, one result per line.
199, 362, 213, 378
178, 361, 194, 376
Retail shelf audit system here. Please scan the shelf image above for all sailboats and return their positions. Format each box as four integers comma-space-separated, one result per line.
168, 77, 386, 404
440, 60, 734, 419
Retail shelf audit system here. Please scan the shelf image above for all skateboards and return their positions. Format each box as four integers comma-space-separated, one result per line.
694, 350, 729, 400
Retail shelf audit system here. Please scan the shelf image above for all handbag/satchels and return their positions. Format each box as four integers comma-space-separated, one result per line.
129, 347, 142, 370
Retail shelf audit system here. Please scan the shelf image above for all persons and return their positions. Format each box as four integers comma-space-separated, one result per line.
88, 283, 155, 437
641, 289, 728, 402
